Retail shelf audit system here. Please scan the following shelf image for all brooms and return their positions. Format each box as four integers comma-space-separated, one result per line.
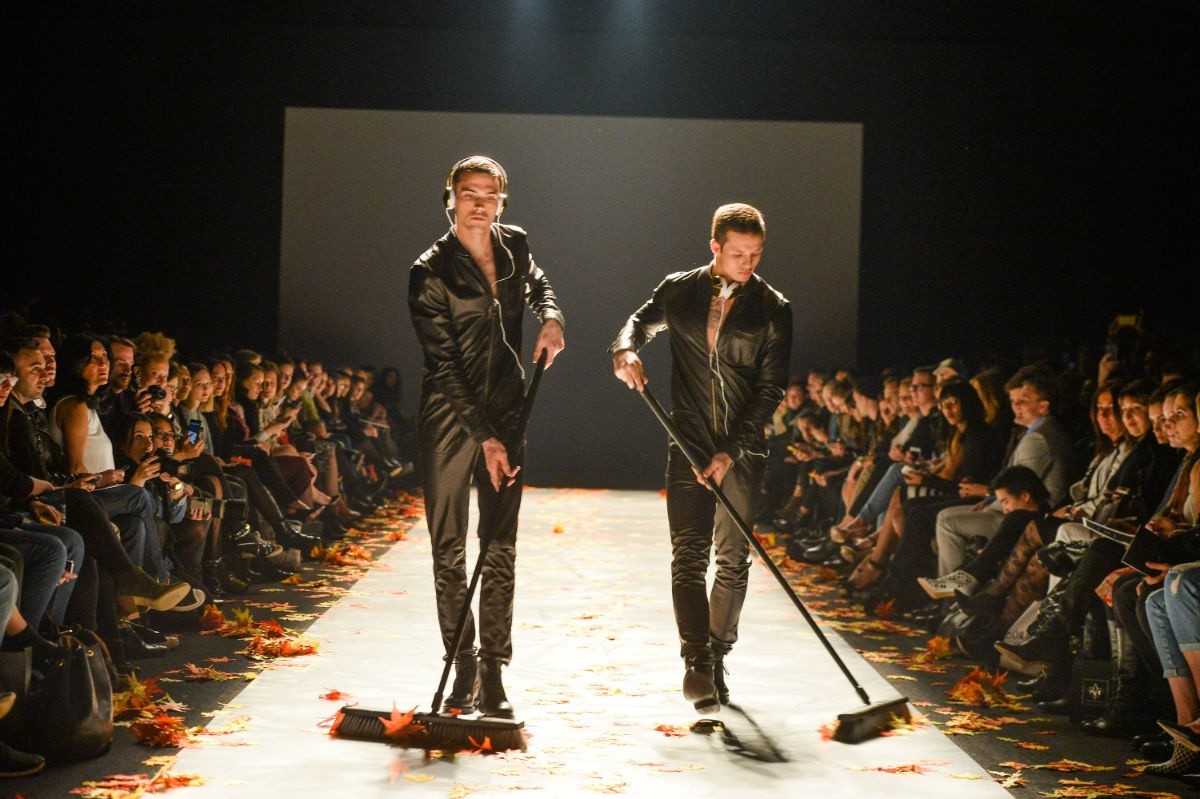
637, 380, 913, 746
327, 348, 547, 754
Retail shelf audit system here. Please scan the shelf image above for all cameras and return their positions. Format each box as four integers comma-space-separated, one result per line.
186, 496, 249, 522
141, 385, 166, 401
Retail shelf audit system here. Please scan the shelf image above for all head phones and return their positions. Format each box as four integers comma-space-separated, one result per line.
707, 260, 748, 300
444, 155, 507, 216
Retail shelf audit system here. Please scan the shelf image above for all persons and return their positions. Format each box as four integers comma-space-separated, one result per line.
758, 351, 1200, 799
608, 204, 795, 715
408, 154, 565, 717
0, 321, 413, 776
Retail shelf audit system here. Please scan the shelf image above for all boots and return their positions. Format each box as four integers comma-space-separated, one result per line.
992, 550, 1114, 703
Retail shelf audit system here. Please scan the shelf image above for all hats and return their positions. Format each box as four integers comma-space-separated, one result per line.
932, 357, 968, 379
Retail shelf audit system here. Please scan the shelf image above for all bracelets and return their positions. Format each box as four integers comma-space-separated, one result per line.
985, 488, 990, 497
167, 482, 185, 493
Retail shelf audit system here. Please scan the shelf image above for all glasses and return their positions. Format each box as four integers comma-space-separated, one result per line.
908, 382, 935, 392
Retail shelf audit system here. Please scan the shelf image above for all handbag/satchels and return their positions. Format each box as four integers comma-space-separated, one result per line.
27, 628, 116, 762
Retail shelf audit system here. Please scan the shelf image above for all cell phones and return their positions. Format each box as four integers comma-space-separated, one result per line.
65, 560, 74, 574
786, 445, 808, 459
910, 447, 921, 463
188, 420, 202, 445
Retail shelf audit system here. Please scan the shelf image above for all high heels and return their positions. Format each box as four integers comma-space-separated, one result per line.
378, 456, 402, 477
112, 566, 206, 622
954, 588, 1005, 627
918, 568, 979, 611
953, 618, 1009, 675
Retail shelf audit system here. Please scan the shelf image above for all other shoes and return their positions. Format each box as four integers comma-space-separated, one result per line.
478, 661, 514, 718
1078, 677, 1152, 737
206, 494, 379, 601
0, 690, 46, 777
116, 622, 180, 659
3, 623, 67, 658
1131, 730, 1200, 774
442, 662, 479, 714
1154, 719, 1199, 752
772, 498, 888, 595
683, 661, 729, 714
